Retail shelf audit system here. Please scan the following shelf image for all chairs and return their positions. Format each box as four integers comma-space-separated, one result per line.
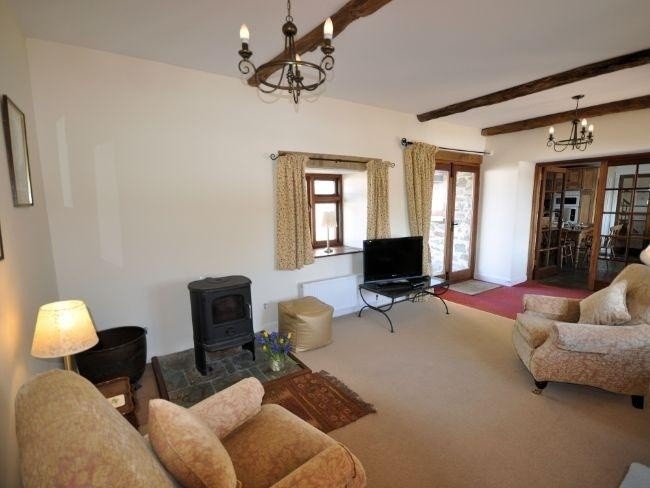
508, 261, 650, 412
536, 215, 626, 277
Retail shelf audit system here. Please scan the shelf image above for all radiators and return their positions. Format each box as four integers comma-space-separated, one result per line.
299, 273, 357, 313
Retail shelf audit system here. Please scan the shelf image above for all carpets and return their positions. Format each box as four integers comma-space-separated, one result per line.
254, 365, 377, 434
442, 278, 504, 297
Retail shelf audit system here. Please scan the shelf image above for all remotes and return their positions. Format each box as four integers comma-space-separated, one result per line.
413, 283, 424, 287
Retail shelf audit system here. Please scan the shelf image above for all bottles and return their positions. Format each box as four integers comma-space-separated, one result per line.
580, 221, 584, 229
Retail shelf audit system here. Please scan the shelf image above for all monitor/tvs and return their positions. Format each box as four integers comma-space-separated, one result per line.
362, 235, 423, 285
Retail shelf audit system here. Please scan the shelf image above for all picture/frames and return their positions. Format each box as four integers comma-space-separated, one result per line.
2, 92, 34, 209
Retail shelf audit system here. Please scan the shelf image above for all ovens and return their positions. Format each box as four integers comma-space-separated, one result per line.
552, 191, 580, 227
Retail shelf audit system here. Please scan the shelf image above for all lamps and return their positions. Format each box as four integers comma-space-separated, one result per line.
28, 297, 100, 373
544, 93, 596, 154
320, 211, 339, 254
235, 1, 340, 106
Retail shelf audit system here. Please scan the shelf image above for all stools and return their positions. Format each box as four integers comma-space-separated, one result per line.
278, 295, 336, 355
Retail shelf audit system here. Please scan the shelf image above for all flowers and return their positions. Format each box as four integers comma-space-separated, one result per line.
251, 330, 299, 373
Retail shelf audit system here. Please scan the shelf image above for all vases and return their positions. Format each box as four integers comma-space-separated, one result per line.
268, 351, 286, 373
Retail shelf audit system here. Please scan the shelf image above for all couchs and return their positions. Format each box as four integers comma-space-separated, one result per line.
13, 368, 369, 487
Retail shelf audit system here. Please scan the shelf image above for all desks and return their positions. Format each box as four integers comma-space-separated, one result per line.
357, 274, 454, 334
90, 374, 142, 432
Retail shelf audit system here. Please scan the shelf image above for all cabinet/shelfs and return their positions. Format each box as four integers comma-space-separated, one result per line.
546, 166, 599, 218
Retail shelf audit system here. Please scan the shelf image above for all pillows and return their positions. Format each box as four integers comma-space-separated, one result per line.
144, 393, 242, 488
575, 279, 632, 328
